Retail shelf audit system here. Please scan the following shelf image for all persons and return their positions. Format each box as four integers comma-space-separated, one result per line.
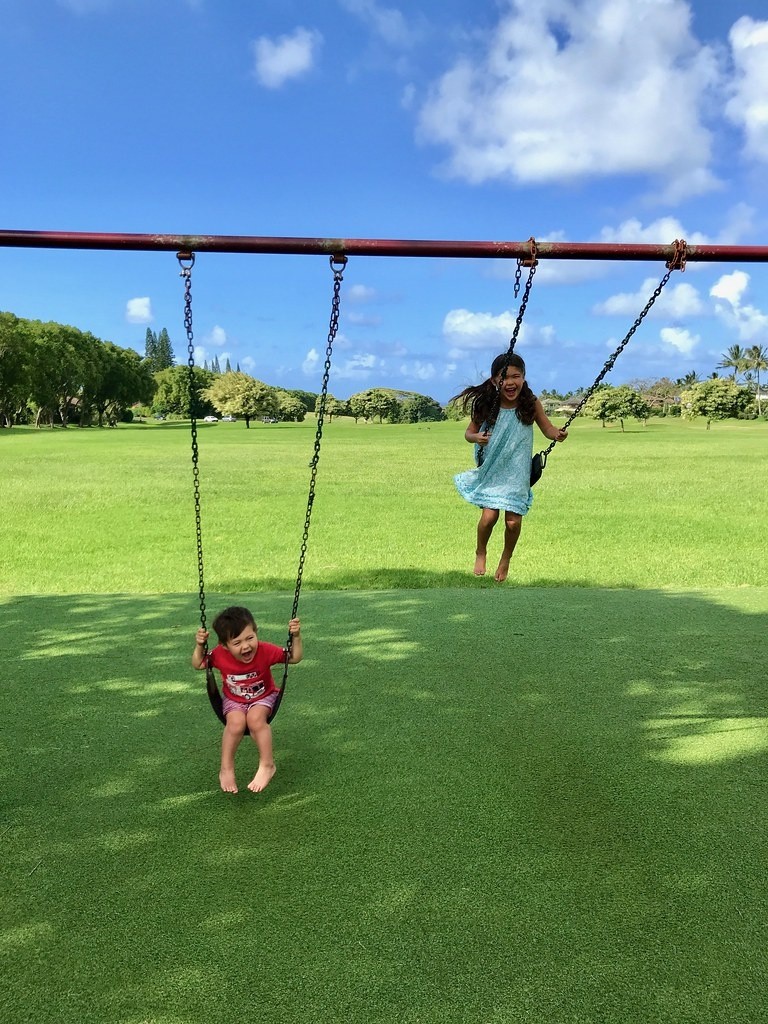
464, 353, 569, 582
192, 606, 302, 794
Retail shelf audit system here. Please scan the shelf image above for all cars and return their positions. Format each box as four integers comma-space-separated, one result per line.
221, 415, 236, 422
262, 416, 279, 424
204, 415, 218, 422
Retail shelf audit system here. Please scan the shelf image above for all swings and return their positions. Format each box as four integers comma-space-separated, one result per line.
477, 263, 675, 487
177, 252, 348, 737
